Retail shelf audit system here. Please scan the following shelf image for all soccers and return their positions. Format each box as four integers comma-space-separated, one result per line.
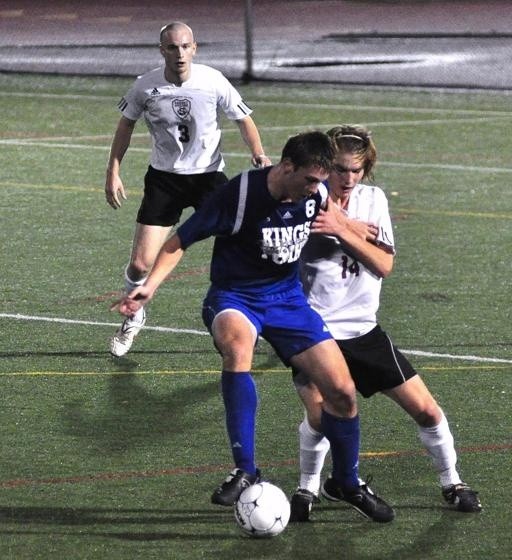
232, 479, 291, 538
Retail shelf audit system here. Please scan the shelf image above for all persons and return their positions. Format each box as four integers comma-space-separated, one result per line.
109, 130, 395, 523
105, 22, 273, 358
286, 126, 482, 523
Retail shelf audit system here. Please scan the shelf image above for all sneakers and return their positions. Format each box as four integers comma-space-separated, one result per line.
211, 467, 262, 507
289, 483, 318, 522
440, 481, 484, 513
110, 309, 147, 358
321, 472, 396, 524
